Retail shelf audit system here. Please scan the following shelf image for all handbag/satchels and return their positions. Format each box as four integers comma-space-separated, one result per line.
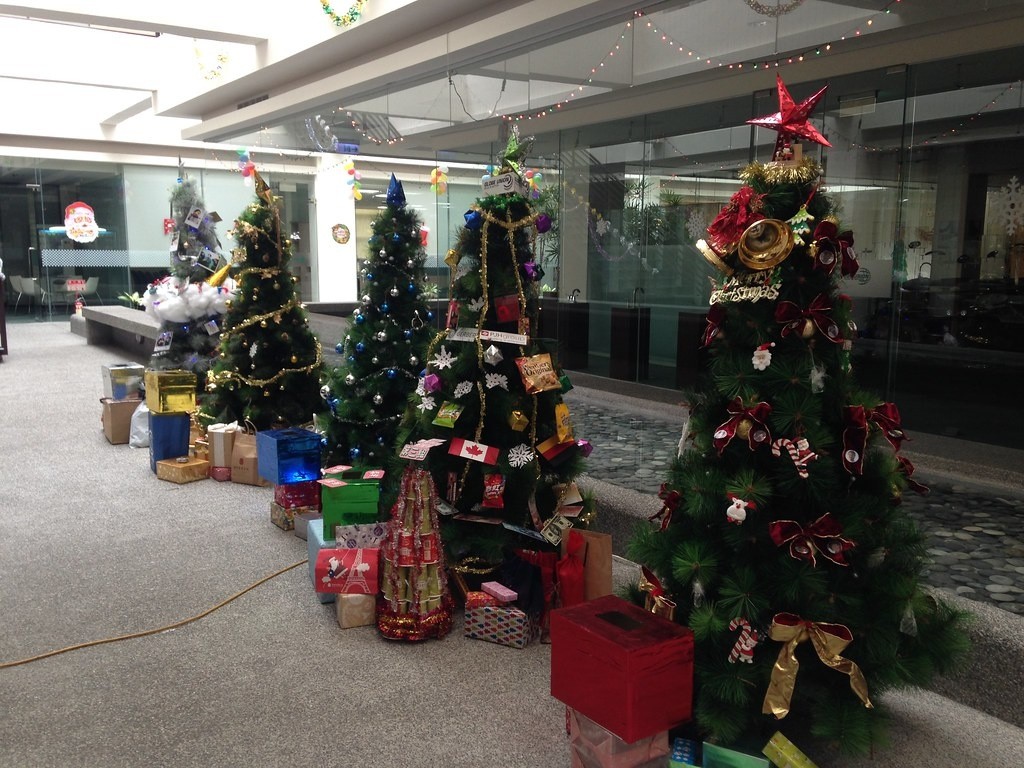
562, 528, 612, 602
231, 420, 270, 487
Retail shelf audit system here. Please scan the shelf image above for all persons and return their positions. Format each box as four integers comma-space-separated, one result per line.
171, 233, 178, 245
198, 251, 218, 270
188, 209, 202, 223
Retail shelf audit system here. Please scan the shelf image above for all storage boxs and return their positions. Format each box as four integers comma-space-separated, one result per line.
335, 593, 377, 629
668, 757, 702, 768
314, 547, 385, 594
703, 724, 775, 768
274, 479, 320, 507
99, 361, 243, 484
306, 518, 336, 603
320, 465, 380, 541
673, 736, 703, 765
549, 595, 695, 744
293, 511, 322, 539
570, 743, 668, 768
467, 591, 507, 607
464, 602, 540, 649
570, 708, 669, 768
62, 279, 87, 291
761, 731, 819, 768
480, 581, 518, 602
269, 503, 319, 530
255, 426, 320, 483
482, 172, 529, 199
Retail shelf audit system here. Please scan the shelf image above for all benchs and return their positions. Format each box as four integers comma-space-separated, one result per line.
83, 305, 163, 344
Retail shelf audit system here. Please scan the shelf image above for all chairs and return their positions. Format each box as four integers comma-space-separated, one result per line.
53, 275, 83, 312
18, 276, 58, 315
9, 275, 31, 314
66, 276, 104, 314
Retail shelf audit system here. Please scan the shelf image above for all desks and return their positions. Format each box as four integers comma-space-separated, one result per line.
54, 290, 87, 313
